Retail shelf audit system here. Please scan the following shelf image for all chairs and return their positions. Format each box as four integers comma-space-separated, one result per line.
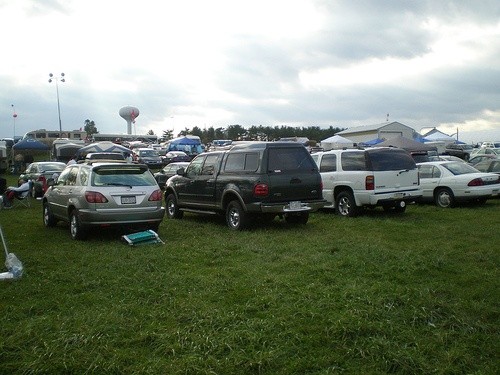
3, 181, 32, 208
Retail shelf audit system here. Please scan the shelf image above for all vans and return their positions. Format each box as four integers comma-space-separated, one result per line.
308, 144, 424, 218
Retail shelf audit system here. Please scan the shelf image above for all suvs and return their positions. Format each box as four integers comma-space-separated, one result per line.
164, 141, 325, 232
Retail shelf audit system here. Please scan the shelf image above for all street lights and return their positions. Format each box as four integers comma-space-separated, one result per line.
49, 73, 65, 137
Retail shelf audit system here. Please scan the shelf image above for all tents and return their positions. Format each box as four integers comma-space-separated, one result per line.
281, 132, 454, 151
12, 136, 52, 174
52, 135, 200, 162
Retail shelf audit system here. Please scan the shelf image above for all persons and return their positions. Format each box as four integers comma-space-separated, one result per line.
14, 151, 34, 173
66, 155, 78, 165
126, 154, 132, 163
2, 176, 30, 209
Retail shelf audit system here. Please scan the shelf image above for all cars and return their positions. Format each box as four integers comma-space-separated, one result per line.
1, 127, 372, 200
428, 141, 500, 173
415, 160, 500, 209
41, 152, 166, 241
